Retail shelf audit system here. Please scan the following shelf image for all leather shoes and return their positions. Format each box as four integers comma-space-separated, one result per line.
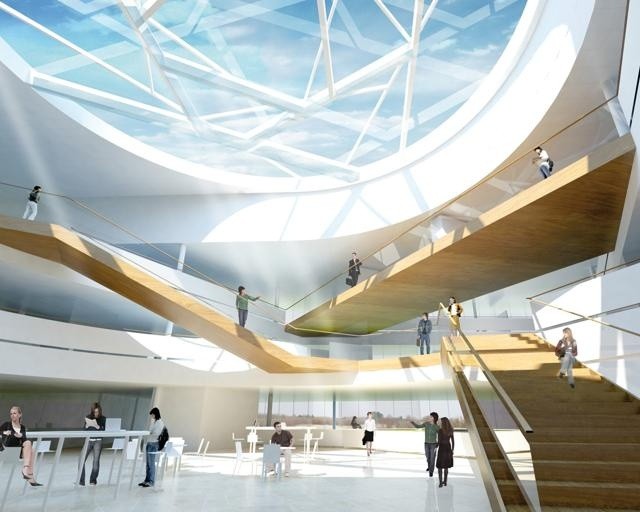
20, 470, 44, 488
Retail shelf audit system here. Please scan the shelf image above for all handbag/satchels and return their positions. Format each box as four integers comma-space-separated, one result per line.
416, 338, 421, 348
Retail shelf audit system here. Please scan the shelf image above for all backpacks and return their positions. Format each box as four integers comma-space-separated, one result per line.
158, 423, 170, 450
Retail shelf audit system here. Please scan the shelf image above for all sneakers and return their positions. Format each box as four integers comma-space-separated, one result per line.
137, 481, 151, 488
266, 469, 291, 477
